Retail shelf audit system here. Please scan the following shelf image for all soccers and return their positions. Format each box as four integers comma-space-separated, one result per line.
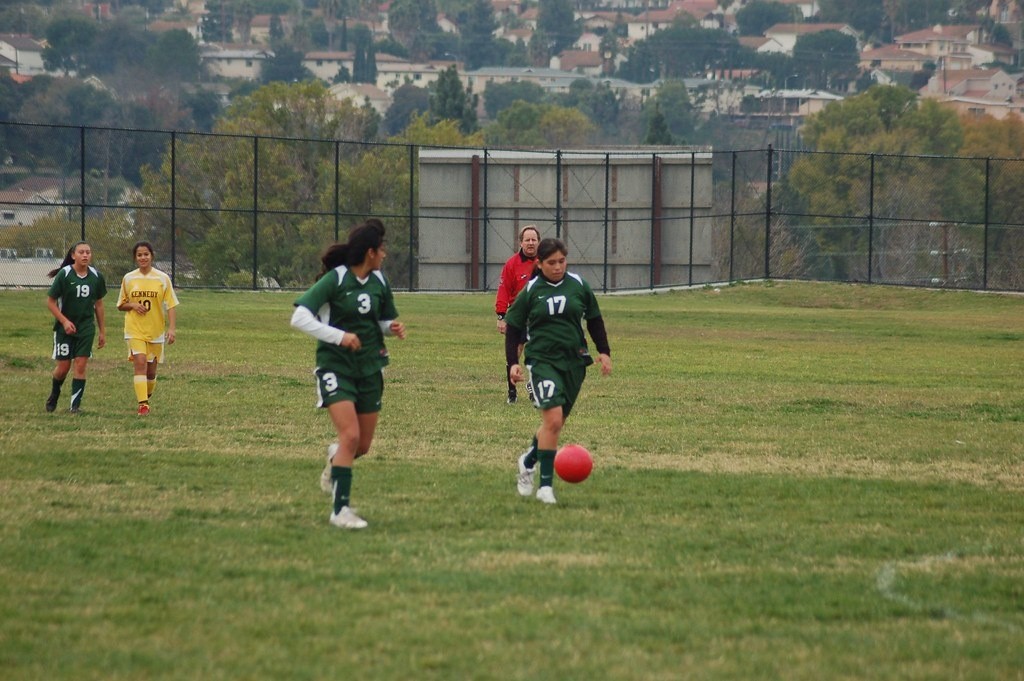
554, 443, 594, 484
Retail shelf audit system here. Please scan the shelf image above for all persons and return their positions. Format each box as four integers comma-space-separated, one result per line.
116, 241, 182, 415
289, 218, 406, 530
46, 241, 108, 412
495, 227, 542, 409
504, 239, 613, 506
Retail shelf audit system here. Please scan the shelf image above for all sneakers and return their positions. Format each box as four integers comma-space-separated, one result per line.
536, 486, 557, 503
70, 406, 79, 412
507, 389, 518, 404
138, 404, 150, 417
517, 453, 536, 495
525, 381, 535, 401
330, 505, 368, 528
46, 391, 61, 412
320, 443, 338, 492
